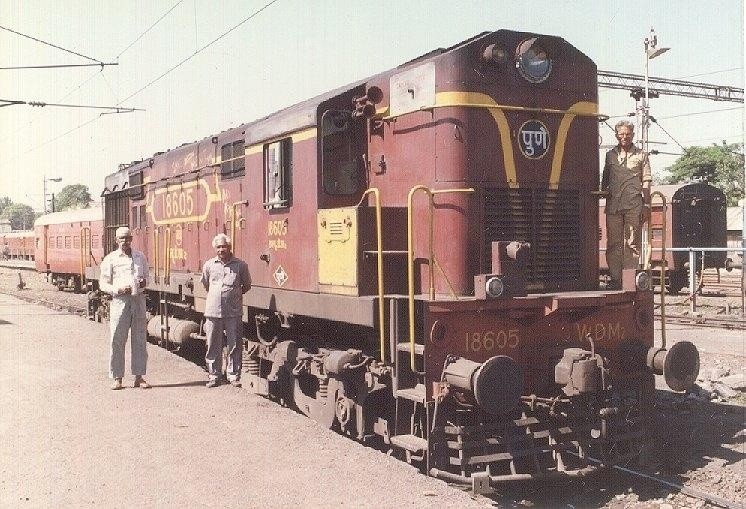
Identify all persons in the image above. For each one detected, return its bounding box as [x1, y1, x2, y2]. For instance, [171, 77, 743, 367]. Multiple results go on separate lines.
[600, 119, 654, 293]
[198, 232, 252, 389]
[98, 226, 152, 389]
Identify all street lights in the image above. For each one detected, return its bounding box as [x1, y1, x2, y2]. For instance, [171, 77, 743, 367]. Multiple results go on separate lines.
[643, 26, 670, 152]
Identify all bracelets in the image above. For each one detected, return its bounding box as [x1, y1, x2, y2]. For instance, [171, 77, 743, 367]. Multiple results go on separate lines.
[644, 203, 652, 209]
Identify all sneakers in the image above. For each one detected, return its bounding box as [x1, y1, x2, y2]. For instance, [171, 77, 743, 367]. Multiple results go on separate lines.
[606, 280, 623, 291]
[132, 380, 152, 389]
[227, 376, 243, 388]
[205, 377, 218, 388]
[110, 378, 122, 390]
